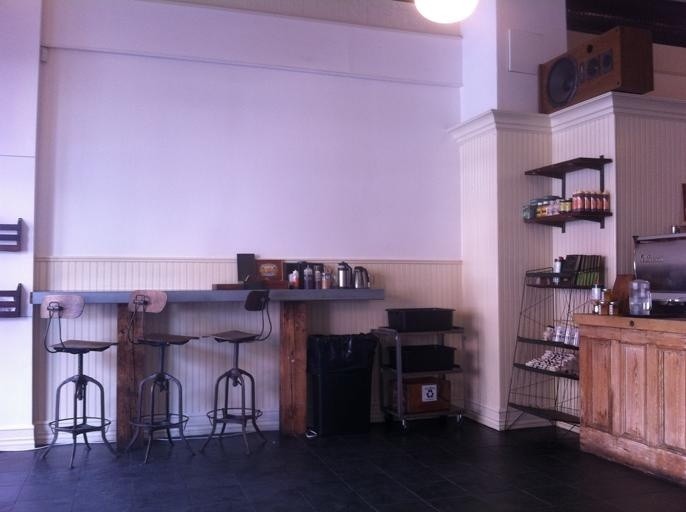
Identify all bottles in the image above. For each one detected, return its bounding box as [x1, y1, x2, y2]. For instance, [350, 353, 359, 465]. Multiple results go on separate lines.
[303, 265, 331, 290]
[591, 283, 618, 316]
[536, 191, 610, 217]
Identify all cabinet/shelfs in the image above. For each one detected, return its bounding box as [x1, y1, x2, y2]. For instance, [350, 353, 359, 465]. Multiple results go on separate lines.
[374, 326, 465, 432]
[523, 154, 613, 234]
[503, 265, 607, 441]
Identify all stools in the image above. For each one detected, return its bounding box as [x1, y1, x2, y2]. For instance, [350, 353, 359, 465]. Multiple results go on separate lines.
[39, 292, 120, 469]
[121, 288, 200, 466]
[199, 290, 271, 457]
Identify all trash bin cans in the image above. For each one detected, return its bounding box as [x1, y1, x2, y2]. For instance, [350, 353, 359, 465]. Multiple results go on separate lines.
[307, 333, 376, 439]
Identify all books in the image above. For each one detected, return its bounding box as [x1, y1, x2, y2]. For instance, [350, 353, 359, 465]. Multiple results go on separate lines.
[558, 254, 601, 286]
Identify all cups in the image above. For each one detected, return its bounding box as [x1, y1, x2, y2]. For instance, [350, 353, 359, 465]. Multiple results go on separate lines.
[628, 280, 652, 316]
[288, 274, 299, 288]
[338, 262, 370, 289]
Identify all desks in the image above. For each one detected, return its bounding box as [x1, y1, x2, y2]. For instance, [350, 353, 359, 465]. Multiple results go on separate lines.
[30, 288, 385, 444]
